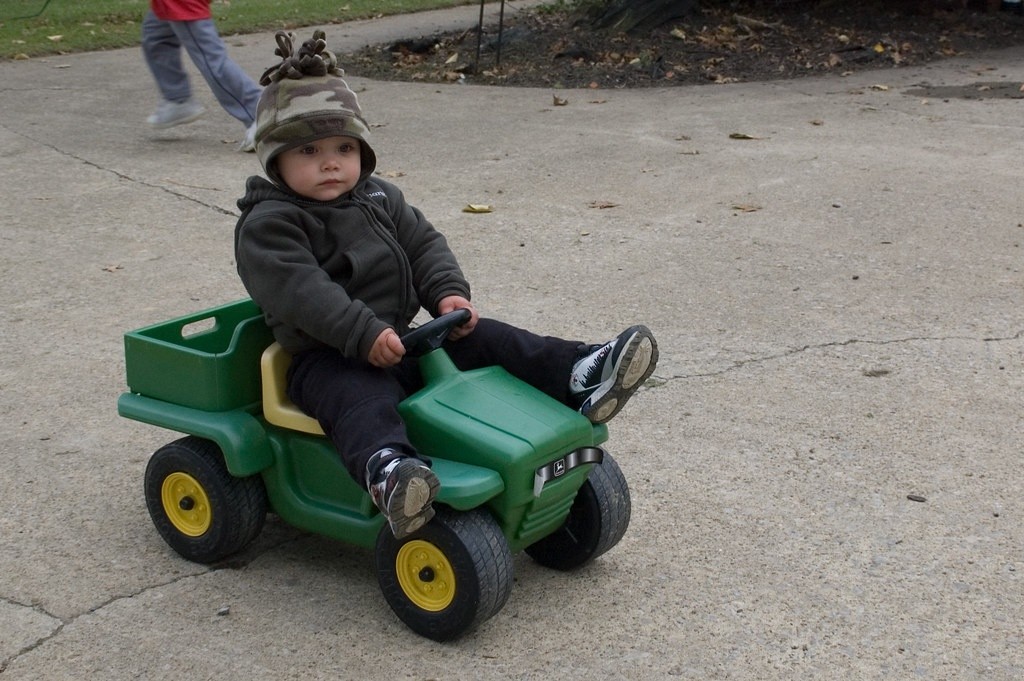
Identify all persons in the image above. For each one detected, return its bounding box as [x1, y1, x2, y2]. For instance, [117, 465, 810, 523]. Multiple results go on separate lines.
[232, 30, 658, 537]
[140, 0, 264, 152]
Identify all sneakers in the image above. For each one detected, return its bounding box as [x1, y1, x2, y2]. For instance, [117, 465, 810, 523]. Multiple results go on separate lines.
[365, 449, 441, 539]
[146, 96, 205, 128]
[243, 122, 257, 153]
[569, 323, 659, 425]
